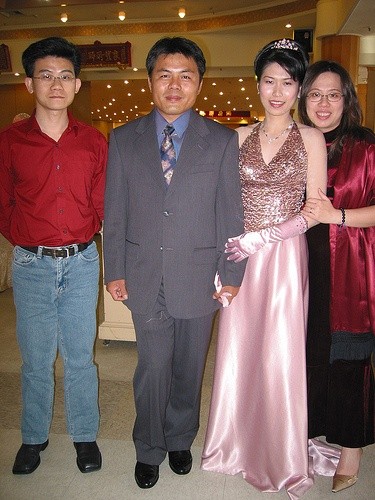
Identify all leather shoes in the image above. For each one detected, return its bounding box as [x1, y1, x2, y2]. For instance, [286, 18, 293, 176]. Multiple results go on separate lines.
[74, 441, 101, 474]
[168, 449, 192, 475]
[12, 439, 48, 475]
[135, 461, 161, 489]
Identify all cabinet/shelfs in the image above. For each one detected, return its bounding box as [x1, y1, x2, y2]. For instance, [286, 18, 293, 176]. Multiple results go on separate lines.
[98, 219, 137, 346]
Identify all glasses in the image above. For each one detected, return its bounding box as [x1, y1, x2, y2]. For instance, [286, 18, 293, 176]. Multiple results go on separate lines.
[305, 92, 345, 102]
[32, 73, 77, 84]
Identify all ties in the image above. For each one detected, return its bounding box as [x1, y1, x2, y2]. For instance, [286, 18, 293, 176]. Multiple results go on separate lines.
[159, 125, 177, 187]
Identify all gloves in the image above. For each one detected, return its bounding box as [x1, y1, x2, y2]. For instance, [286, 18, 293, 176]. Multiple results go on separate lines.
[224, 213, 308, 264]
[214, 271, 232, 308]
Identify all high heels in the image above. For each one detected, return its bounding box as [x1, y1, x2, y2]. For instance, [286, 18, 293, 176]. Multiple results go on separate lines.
[331, 447, 363, 492]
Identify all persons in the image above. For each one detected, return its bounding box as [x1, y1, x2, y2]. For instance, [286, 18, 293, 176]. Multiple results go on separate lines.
[297, 60, 375, 493]
[0, 36, 108, 474]
[104, 37, 248, 488]
[213, 39, 327, 500]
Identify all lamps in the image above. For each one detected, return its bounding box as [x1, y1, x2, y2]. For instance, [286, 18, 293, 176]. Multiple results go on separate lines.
[119, 10, 125, 21]
[178, 8, 186, 19]
[60, 13, 68, 23]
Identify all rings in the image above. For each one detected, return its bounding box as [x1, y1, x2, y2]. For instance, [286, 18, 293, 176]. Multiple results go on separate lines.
[310, 208, 312, 213]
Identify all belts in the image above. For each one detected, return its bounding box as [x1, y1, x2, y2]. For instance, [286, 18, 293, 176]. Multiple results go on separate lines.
[18, 238, 96, 259]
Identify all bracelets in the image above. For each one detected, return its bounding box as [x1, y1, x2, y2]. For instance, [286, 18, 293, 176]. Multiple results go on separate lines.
[337, 207, 345, 227]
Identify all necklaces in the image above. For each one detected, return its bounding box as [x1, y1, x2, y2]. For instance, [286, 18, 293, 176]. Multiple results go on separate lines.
[263, 123, 295, 144]
